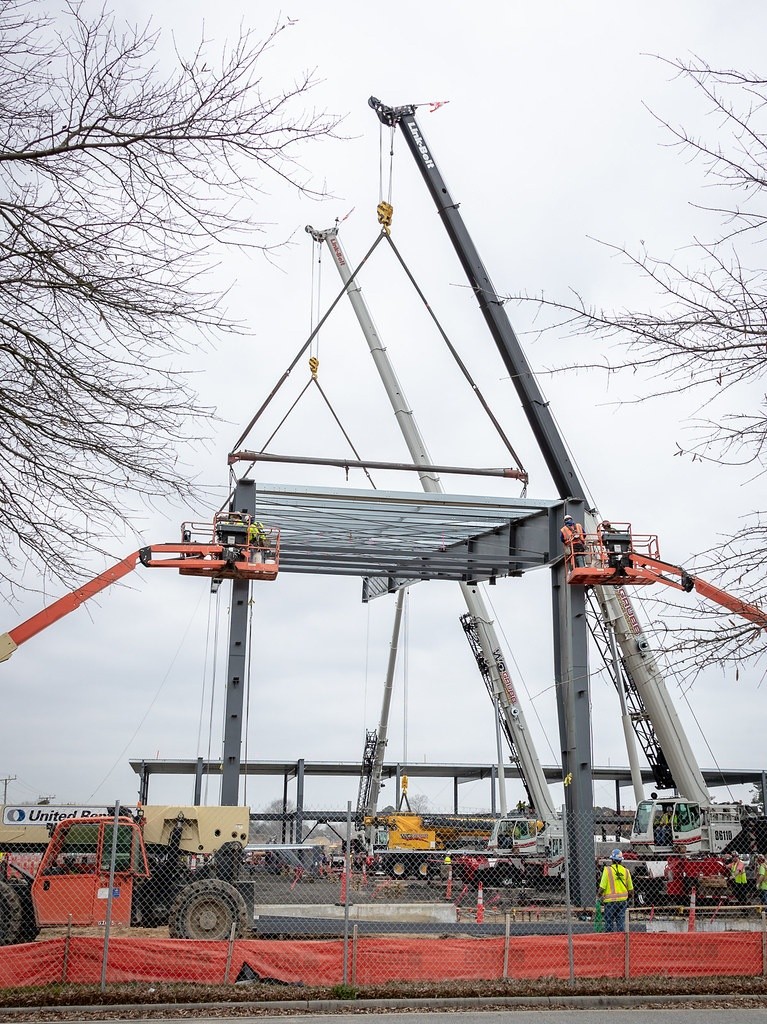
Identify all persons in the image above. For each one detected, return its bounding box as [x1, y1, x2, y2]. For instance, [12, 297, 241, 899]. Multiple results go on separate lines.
[559, 515, 587, 572]
[601, 826, 607, 842]
[595, 848, 634, 933]
[602, 520, 637, 553]
[723, 851, 750, 919]
[655, 807, 677, 846]
[500, 825, 520, 849]
[516, 800, 526, 814]
[755, 853, 767, 916]
[615, 825, 622, 842]
[228, 511, 268, 547]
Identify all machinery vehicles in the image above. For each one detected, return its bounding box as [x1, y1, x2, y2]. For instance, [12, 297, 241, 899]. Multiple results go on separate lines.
[0, 798, 258, 950]
[354, 812, 452, 881]
[300, 95, 767, 921]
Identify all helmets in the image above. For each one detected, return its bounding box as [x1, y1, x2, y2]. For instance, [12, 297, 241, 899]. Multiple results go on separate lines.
[731, 851, 739, 857]
[609, 849, 624, 861]
[232, 511, 242, 519]
[666, 806, 673, 812]
[245, 516, 253, 521]
[758, 854, 765, 861]
